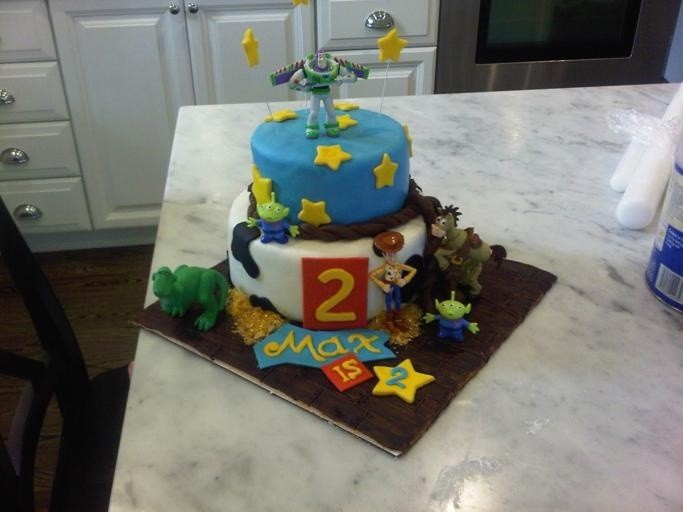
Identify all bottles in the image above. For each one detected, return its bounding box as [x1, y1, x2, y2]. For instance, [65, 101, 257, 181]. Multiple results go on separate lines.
[644, 132, 683, 313]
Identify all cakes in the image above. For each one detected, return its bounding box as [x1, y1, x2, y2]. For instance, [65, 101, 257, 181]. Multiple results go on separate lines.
[226, 50, 442, 323]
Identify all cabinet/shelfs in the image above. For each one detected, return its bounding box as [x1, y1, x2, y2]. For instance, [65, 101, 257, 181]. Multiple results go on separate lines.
[316, 2, 443, 98]
[0, 0, 93, 253]
[43, 1, 315, 245]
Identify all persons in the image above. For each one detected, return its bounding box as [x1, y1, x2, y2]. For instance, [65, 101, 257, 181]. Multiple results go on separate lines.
[369, 230, 417, 333]
[269, 47, 371, 139]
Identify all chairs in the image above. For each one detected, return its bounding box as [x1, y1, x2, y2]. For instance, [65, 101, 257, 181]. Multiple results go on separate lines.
[0, 196, 134, 512]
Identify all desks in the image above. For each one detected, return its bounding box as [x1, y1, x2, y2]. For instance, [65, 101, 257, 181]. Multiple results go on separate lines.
[102, 82, 682, 512]
